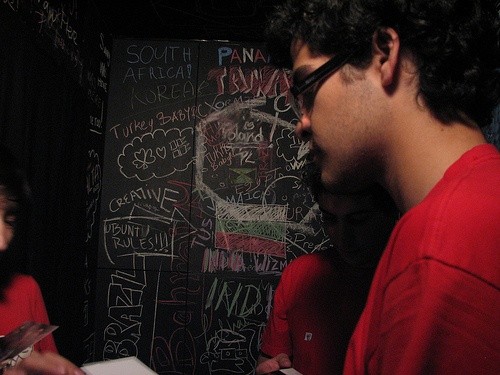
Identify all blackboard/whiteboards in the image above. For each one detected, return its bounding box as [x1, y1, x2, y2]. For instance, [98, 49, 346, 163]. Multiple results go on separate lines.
[94, 38, 333, 375]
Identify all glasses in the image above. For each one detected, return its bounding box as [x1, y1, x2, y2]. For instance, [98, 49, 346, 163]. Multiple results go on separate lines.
[288, 39, 365, 118]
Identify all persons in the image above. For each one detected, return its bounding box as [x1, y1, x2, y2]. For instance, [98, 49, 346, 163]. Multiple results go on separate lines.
[267, 0, 500, 375]
[0, 146, 90, 375]
[255, 164, 402, 375]
